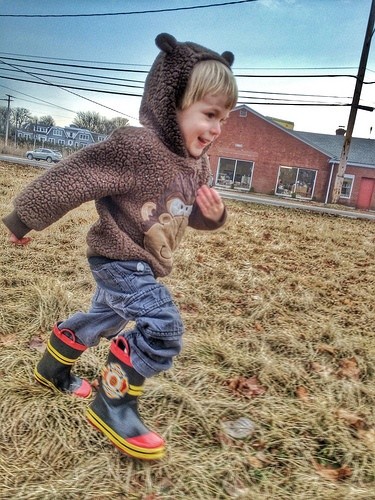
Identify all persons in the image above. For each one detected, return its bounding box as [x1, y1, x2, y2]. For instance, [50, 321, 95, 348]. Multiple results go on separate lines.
[2, 32, 240, 458]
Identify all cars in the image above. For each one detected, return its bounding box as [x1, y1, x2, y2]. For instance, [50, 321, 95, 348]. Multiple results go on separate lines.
[27, 145, 64, 164]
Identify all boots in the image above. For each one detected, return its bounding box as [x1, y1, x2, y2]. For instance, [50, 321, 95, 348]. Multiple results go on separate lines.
[33, 321, 93, 401]
[84, 335, 168, 461]
